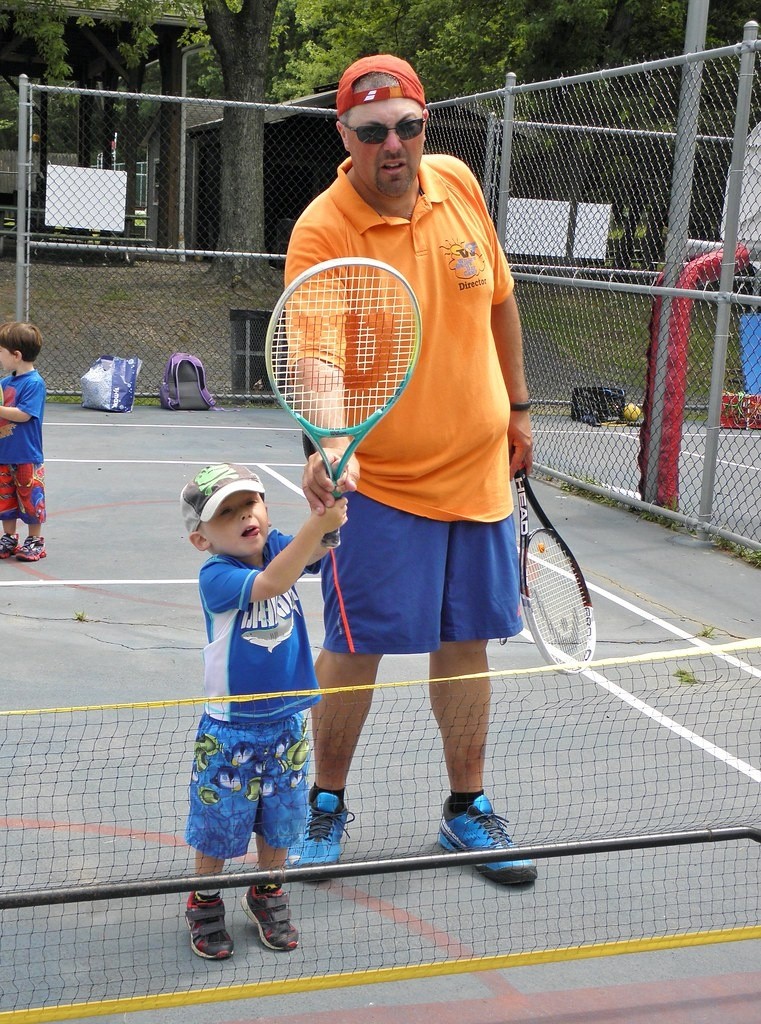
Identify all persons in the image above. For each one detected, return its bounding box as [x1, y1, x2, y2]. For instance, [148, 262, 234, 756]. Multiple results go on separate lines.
[0, 321, 47, 563]
[179, 456, 352, 958]
[281, 54, 539, 886]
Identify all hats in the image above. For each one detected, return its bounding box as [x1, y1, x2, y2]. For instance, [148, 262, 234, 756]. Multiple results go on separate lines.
[336, 53, 427, 110]
[179, 462, 266, 534]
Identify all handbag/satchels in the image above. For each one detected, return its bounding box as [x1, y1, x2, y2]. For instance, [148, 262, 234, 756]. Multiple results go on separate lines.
[81, 356, 143, 413]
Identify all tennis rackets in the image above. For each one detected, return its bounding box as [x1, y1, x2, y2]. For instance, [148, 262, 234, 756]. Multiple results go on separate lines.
[265, 255, 423, 549]
[514, 461, 596, 676]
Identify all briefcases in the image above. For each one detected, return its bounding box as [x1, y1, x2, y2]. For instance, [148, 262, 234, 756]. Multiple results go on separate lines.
[717, 390, 760, 428]
[572, 384, 625, 424]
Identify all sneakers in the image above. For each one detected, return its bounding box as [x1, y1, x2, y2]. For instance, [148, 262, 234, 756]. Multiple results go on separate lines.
[15, 536, 46, 561]
[186, 890, 233, 959]
[239, 881, 300, 952]
[438, 795, 538, 883]
[0, 532, 18, 558]
[288, 793, 349, 866]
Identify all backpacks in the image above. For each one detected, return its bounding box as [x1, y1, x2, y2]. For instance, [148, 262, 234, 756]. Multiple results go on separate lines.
[161, 353, 215, 410]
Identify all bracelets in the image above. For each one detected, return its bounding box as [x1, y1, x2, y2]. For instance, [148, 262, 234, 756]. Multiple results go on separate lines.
[510, 398, 532, 411]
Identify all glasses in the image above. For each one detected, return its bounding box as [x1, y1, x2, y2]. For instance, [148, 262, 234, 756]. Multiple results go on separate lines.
[341, 117, 425, 144]
